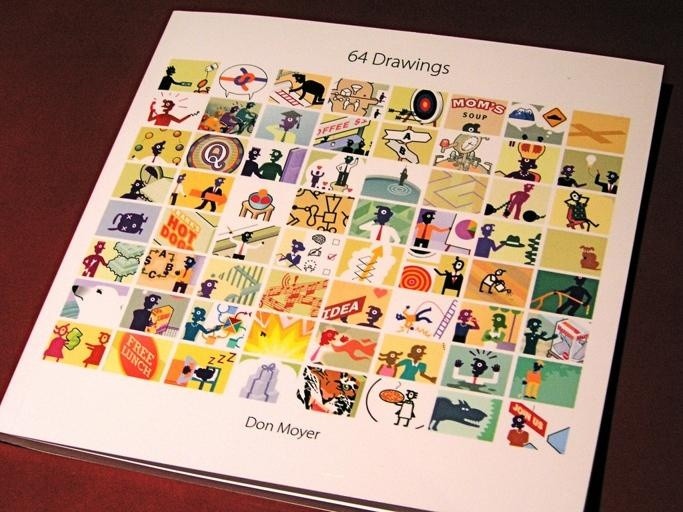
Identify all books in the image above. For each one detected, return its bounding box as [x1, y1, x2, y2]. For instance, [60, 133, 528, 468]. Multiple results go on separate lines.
[0, 12, 664, 512]
